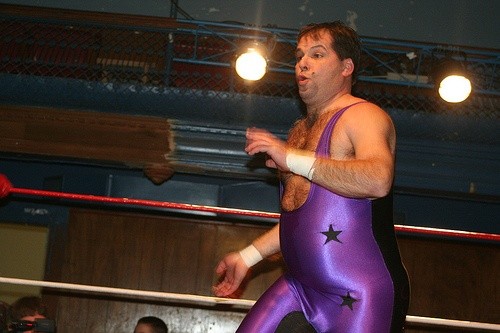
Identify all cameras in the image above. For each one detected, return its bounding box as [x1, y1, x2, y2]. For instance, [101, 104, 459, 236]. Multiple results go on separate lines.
[14, 319, 55, 333]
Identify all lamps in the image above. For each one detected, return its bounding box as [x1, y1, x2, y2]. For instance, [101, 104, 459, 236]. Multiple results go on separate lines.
[230, 27, 474, 105]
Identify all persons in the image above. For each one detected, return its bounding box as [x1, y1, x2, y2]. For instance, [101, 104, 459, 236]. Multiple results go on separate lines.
[7, 296, 50, 333]
[132, 315, 168, 333]
[211, 20, 410, 333]
